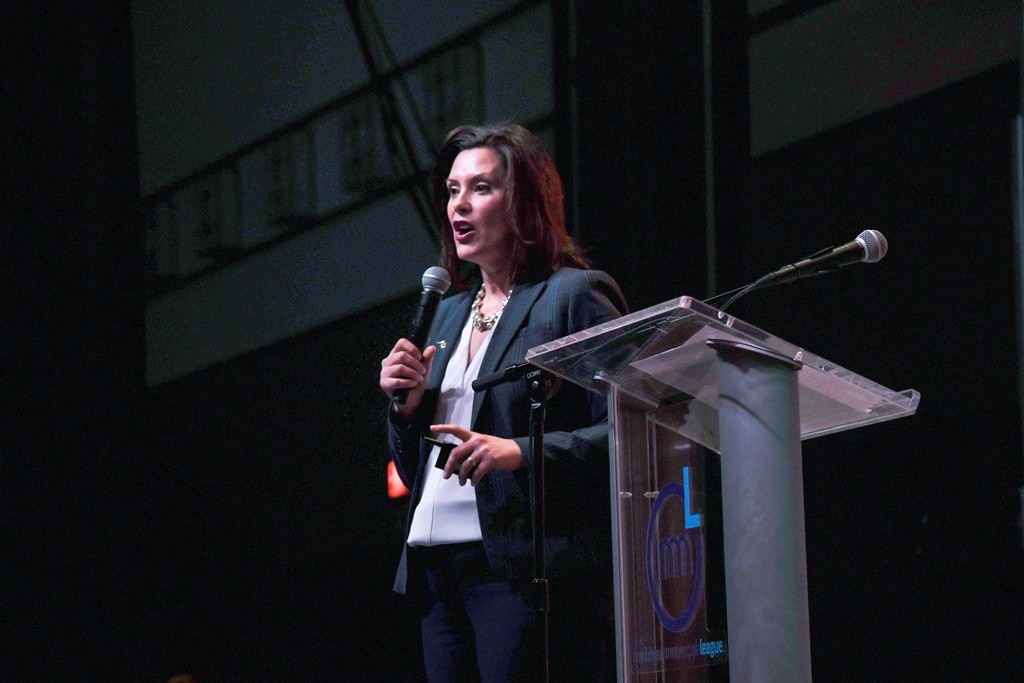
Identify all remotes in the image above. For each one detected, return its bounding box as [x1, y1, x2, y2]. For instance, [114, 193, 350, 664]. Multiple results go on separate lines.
[424, 437, 478, 478]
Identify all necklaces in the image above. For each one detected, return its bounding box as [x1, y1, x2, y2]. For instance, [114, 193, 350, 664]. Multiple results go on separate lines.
[471, 283, 513, 332]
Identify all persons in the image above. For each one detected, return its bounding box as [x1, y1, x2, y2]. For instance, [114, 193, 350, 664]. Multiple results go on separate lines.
[380, 124, 644, 683]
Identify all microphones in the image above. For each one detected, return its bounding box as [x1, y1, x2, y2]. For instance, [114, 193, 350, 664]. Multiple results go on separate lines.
[394, 266, 451, 406]
[750, 229, 888, 290]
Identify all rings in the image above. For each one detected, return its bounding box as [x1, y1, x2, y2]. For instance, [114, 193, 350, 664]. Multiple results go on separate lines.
[468, 457, 476, 467]
